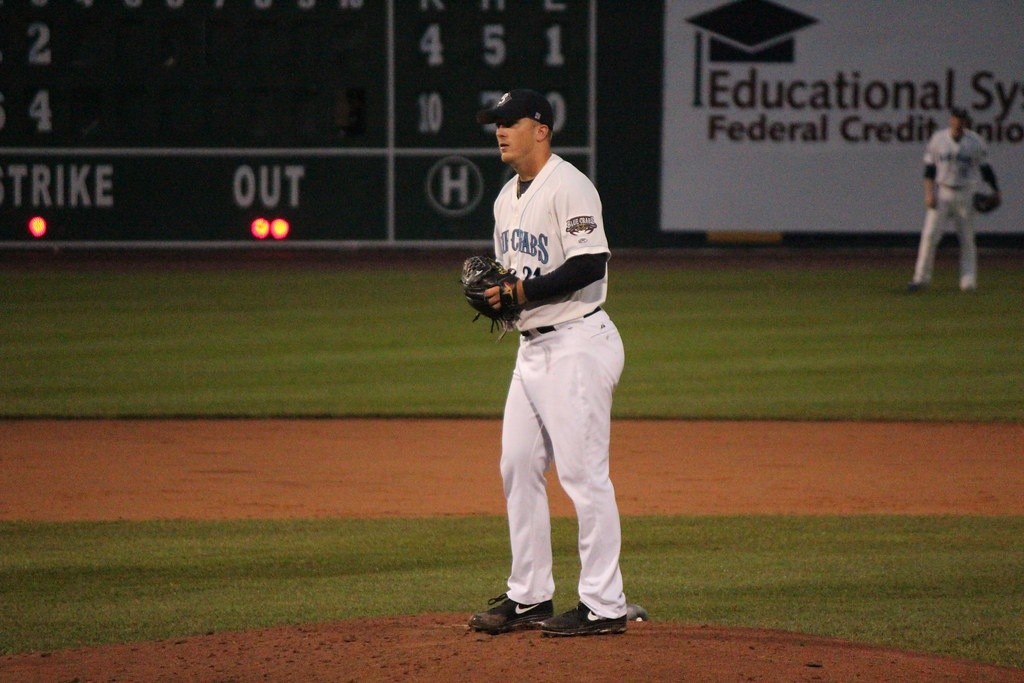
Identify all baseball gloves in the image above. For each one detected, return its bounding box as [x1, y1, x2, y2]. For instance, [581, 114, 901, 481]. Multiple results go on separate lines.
[972, 191, 1001, 215]
[460, 254, 509, 318]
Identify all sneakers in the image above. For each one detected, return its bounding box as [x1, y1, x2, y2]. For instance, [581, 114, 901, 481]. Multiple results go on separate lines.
[539, 602, 628, 637]
[468, 599, 554, 634]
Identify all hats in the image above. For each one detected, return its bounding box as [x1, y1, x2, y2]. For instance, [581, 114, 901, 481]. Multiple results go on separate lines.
[477, 88, 554, 131]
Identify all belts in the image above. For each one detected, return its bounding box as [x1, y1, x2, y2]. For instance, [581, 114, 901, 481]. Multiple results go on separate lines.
[521, 306, 602, 339]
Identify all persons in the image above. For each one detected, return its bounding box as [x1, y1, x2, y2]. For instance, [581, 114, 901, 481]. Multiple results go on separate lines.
[906, 106, 1002, 296]
[459, 89, 627, 637]
